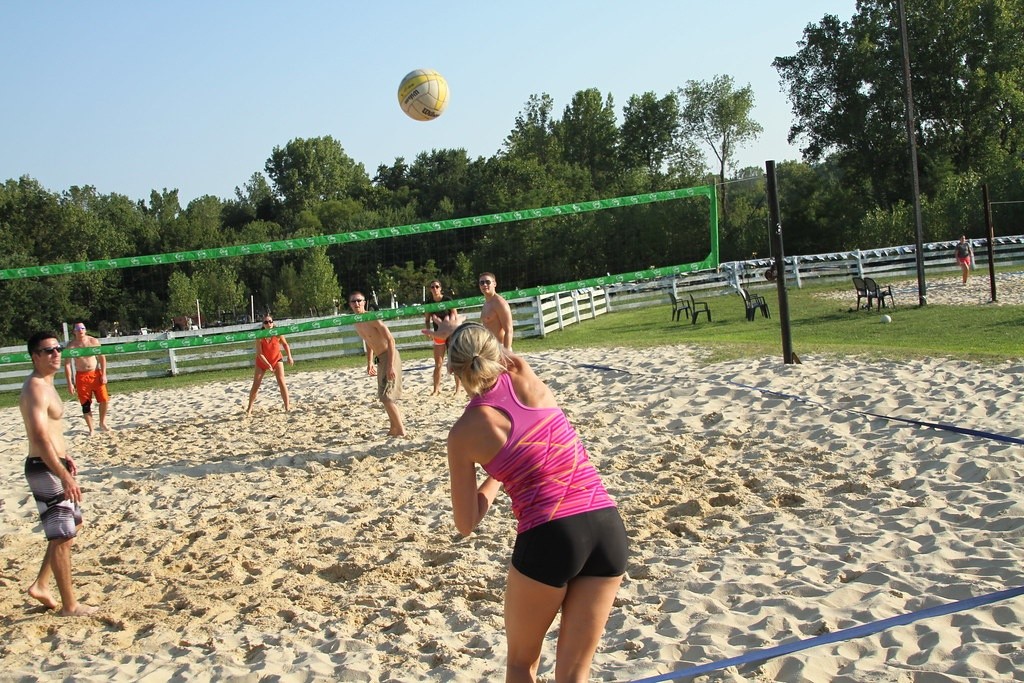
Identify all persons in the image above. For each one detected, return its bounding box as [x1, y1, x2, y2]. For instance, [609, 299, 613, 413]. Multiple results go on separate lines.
[19, 331, 100, 617]
[246, 313, 293, 413]
[416, 310, 628, 683]
[423, 280, 460, 395]
[64, 323, 108, 432]
[477, 272, 513, 354]
[955, 235, 973, 285]
[349, 291, 404, 439]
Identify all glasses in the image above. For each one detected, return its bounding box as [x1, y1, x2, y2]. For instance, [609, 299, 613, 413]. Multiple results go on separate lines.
[35, 345, 62, 354]
[431, 285, 440, 288]
[264, 320, 273, 324]
[480, 280, 493, 284]
[73, 326, 86, 331]
[351, 299, 363, 303]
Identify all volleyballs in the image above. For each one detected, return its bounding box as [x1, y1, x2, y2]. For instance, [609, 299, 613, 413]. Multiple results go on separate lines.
[397, 68, 450, 122]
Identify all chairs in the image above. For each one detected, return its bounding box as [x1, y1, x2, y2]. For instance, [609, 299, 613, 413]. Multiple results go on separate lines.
[668, 292, 692, 322]
[689, 293, 712, 324]
[852, 277, 895, 311]
[739, 289, 772, 321]
